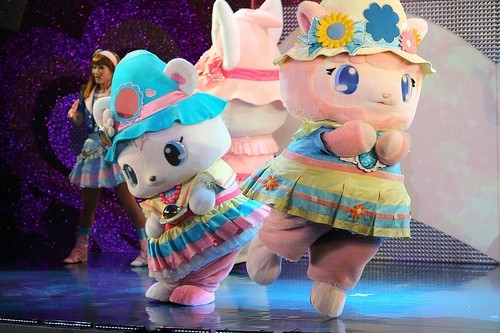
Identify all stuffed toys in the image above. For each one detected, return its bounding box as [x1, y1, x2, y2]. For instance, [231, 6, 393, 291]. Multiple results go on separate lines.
[237, 1, 437, 317]
[194, 0, 287, 266]
[93, 50, 273, 306]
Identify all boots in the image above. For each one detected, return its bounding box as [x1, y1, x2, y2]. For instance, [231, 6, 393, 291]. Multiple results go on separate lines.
[64, 226, 92, 263]
[130, 228, 148, 266]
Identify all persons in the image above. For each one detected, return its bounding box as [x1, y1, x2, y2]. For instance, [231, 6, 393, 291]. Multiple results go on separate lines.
[64, 49, 146, 266]
